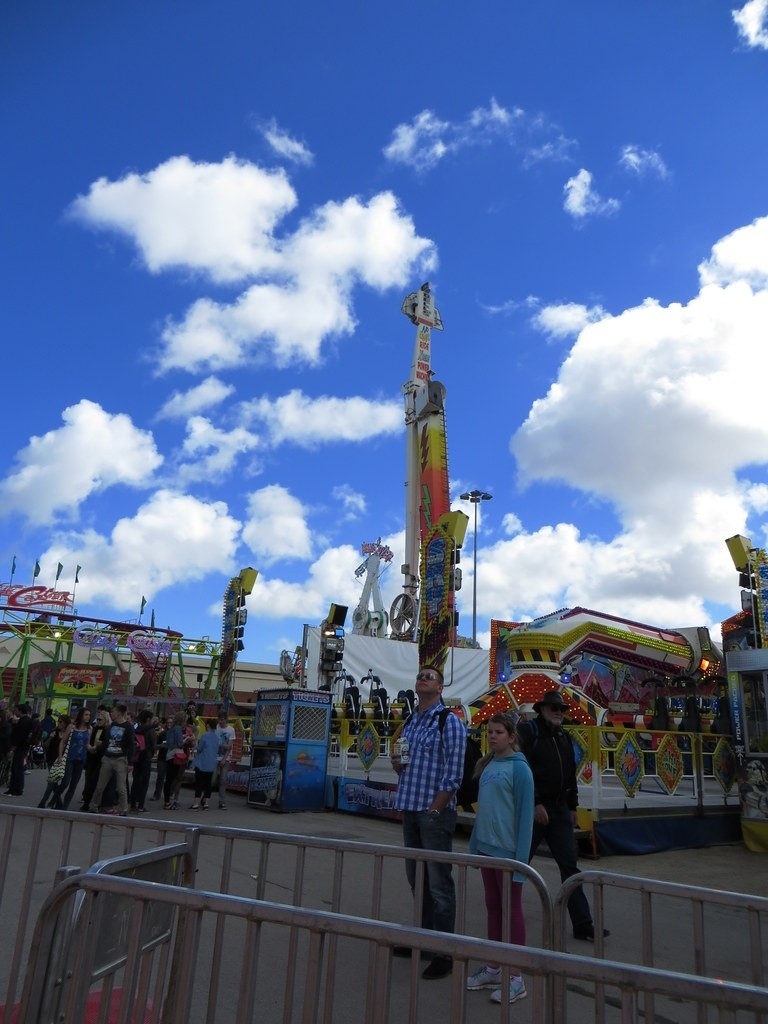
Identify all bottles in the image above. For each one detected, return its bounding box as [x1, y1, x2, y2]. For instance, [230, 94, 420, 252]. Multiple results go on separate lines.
[107, 747, 115, 753]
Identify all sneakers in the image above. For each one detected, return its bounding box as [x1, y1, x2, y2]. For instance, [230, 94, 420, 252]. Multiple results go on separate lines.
[130, 808, 151, 815]
[80, 804, 90, 810]
[219, 802, 227, 810]
[490, 976, 527, 1003]
[422, 954, 454, 980]
[119, 809, 127, 816]
[202, 805, 208, 813]
[2, 790, 24, 798]
[38, 803, 46, 808]
[148, 797, 161, 804]
[164, 803, 178, 810]
[88, 806, 98, 813]
[393, 939, 432, 960]
[467, 965, 502, 989]
[187, 804, 199, 813]
[24, 769, 31, 774]
[573, 921, 611, 939]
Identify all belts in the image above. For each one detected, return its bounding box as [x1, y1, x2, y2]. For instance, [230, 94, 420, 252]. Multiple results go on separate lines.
[105, 750, 124, 758]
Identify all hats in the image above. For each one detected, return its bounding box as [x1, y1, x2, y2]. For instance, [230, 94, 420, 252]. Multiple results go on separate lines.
[532, 692, 571, 712]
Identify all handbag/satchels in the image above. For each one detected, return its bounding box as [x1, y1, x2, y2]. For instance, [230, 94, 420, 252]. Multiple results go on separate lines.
[33, 744, 43, 754]
[174, 753, 187, 765]
[47, 727, 73, 783]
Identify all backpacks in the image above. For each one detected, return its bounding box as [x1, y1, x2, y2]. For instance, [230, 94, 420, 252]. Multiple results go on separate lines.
[132, 733, 145, 763]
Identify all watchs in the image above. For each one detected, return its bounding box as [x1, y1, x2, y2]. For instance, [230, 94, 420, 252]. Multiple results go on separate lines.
[429, 809, 440, 819]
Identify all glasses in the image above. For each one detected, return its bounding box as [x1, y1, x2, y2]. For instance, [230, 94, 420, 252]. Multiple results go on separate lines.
[416, 673, 441, 680]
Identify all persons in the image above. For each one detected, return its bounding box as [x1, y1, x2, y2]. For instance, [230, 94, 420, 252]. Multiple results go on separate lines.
[467, 711, 534, 1003]
[0, 700, 236, 816]
[711, 698, 732, 743]
[516, 691, 610, 941]
[678, 697, 701, 752]
[648, 697, 670, 731]
[390, 665, 468, 979]
